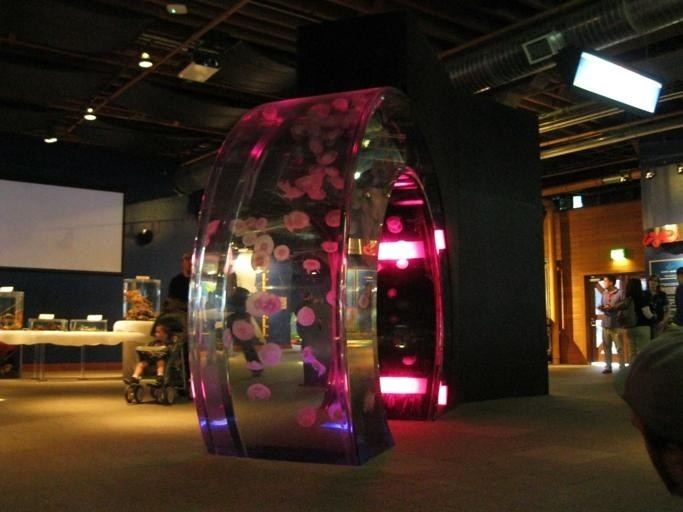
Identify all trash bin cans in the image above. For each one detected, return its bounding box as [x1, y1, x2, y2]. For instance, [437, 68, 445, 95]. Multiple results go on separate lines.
[546, 317, 552, 364]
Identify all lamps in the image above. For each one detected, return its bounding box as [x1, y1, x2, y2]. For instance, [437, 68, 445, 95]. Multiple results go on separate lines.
[558, 47, 665, 120]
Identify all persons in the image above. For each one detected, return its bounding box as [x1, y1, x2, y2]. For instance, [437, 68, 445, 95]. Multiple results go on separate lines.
[596, 267, 683, 374]
[610, 329, 683, 499]
[164, 250, 200, 312]
[222, 272, 264, 379]
[122, 321, 176, 389]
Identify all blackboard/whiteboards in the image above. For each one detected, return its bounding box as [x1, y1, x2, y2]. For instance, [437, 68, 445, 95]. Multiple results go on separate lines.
[0, 178, 125, 275]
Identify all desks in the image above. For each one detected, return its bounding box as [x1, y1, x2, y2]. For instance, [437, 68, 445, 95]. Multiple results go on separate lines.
[0, 328, 146, 382]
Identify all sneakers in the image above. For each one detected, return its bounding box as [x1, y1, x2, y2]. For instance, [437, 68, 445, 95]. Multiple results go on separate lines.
[156, 376, 164, 384]
[123, 377, 139, 384]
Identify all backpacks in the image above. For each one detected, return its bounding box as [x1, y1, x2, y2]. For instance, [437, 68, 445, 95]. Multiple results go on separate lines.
[617, 297, 637, 327]
